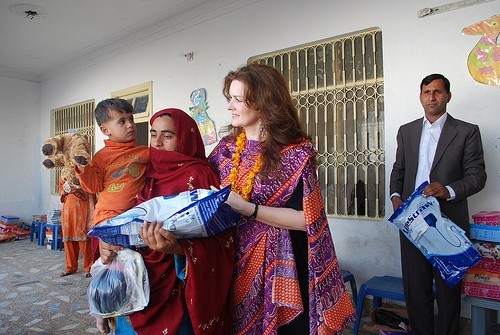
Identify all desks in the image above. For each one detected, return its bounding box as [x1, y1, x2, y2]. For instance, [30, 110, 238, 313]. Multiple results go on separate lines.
[462, 296, 500, 335]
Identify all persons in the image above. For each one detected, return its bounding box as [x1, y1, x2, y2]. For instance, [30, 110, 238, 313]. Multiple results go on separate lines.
[209, 64, 359, 335]
[99, 108, 236, 335]
[389, 73, 487, 335]
[72, 97, 149, 335]
[59, 168, 99, 278]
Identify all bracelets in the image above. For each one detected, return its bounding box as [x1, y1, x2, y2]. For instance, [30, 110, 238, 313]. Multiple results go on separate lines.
[250, 203, 258, 219]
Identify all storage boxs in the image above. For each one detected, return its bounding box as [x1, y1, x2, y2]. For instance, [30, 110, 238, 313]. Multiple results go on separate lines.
[45, 231, 53, 240]
[0, 221, 17, 229]
[463, 242, 500, 300]
[47, 242, 64, 250]
[471, 208, 500, 226]
[469, 224, 500, 243]
[1, 215, 20, 223]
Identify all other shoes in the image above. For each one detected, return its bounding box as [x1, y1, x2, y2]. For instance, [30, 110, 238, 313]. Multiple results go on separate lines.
[60, 271, 72, 276]
[85, 273, 91, 278]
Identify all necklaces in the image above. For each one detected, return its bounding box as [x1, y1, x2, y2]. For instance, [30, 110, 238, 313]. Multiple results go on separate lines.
[230, 130, 263, 200]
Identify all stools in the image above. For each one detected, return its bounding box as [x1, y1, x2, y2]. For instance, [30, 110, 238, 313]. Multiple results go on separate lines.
[341, 267, 358, 306]
[40, 222, 64, 251]
[29, 220, 41, 244]
[352, 275, 410, 335]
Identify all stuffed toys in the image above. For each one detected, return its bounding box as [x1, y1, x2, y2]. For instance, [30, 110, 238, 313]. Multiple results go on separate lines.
[42, 133, 90, 167]
